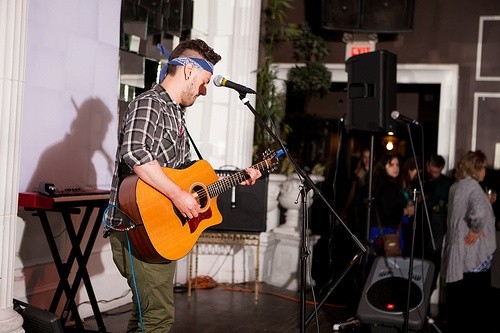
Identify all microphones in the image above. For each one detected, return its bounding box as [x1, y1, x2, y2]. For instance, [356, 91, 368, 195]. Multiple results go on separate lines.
[391, 111, 418, 126]
[213, 75, 257, 94]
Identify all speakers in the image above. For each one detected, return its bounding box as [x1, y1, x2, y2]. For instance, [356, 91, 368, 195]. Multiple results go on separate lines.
[205, 170, 269, 232]
[344, 49, 398, 133]
[146, 0, 194, 37]
[357, 256, 435, 332]
[321, 0, 361, 32]
[361, 0, 415, 33]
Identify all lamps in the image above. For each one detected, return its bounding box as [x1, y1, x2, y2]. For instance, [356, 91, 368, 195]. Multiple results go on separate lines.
[342, 32, 378, 60]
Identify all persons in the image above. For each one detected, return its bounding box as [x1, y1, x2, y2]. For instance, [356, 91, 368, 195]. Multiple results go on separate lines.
[409, 155, 452, 267]
[339, 149, 375, 237]
[103, 39, 262, 333]
[442, 150, 497, 333]
[369, 154, 414, 257]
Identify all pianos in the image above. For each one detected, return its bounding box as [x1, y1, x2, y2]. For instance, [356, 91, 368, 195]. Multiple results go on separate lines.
[18, 185, 111, 333]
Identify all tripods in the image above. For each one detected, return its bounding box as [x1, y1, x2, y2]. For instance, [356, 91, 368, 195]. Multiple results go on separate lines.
[298, 135, 375, 327]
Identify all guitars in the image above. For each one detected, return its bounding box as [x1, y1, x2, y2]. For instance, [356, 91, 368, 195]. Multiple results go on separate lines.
[118, 148, 281, 263]
